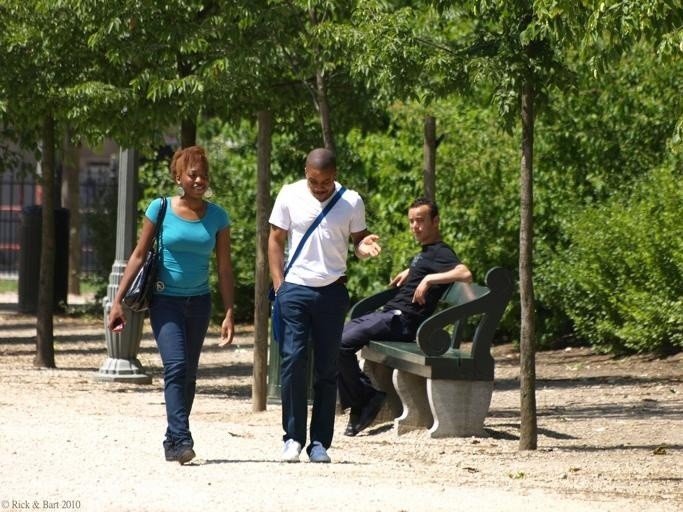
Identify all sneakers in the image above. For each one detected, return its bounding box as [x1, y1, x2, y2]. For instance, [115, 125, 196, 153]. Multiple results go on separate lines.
[345, 388, 386, 436]
[309, 441, 331, 463]
[164, 447, 195, 465]
[282, 438, 301, 461]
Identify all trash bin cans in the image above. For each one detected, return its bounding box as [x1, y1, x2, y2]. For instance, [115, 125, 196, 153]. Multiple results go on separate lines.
[18, 205, 71, 314]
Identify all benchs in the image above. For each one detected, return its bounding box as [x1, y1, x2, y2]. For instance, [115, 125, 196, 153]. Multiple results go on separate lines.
[358, 266, 512, 440]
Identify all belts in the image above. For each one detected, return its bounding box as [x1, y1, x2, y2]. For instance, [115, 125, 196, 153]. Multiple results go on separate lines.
[388, 308, 405, 314]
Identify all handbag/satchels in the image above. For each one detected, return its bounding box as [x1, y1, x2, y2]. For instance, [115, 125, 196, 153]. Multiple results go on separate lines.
[270, 296, 284, 343]
[122, 195, 166, 312]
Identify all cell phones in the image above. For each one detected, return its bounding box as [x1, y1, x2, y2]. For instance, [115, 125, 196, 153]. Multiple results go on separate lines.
[112, 319, 123, 332]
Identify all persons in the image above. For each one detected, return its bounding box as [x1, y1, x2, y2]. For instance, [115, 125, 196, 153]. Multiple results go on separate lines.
[266, 147, 381, 465]
[103, 147, 235, 466]
[336, 197, 474, 437]
